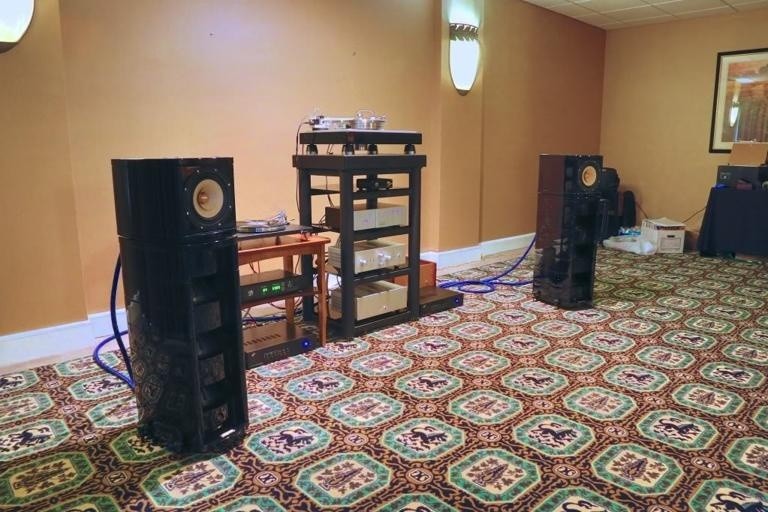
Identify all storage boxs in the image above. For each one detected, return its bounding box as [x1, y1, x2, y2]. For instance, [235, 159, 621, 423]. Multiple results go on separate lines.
[640, 216, 686, 254]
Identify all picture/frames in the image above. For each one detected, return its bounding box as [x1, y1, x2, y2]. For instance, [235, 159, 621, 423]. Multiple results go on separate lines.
[709, 48, 768, 153]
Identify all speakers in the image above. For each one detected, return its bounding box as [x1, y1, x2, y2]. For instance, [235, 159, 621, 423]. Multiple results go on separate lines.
[533, 154, 603, 309]
[110, 156, 249, 454]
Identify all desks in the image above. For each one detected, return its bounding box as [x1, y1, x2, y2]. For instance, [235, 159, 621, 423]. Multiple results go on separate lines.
[236, 232, 332, 347]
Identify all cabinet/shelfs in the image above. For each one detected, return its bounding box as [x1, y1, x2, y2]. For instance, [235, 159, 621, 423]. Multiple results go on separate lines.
[292, 152, 427, 339]
